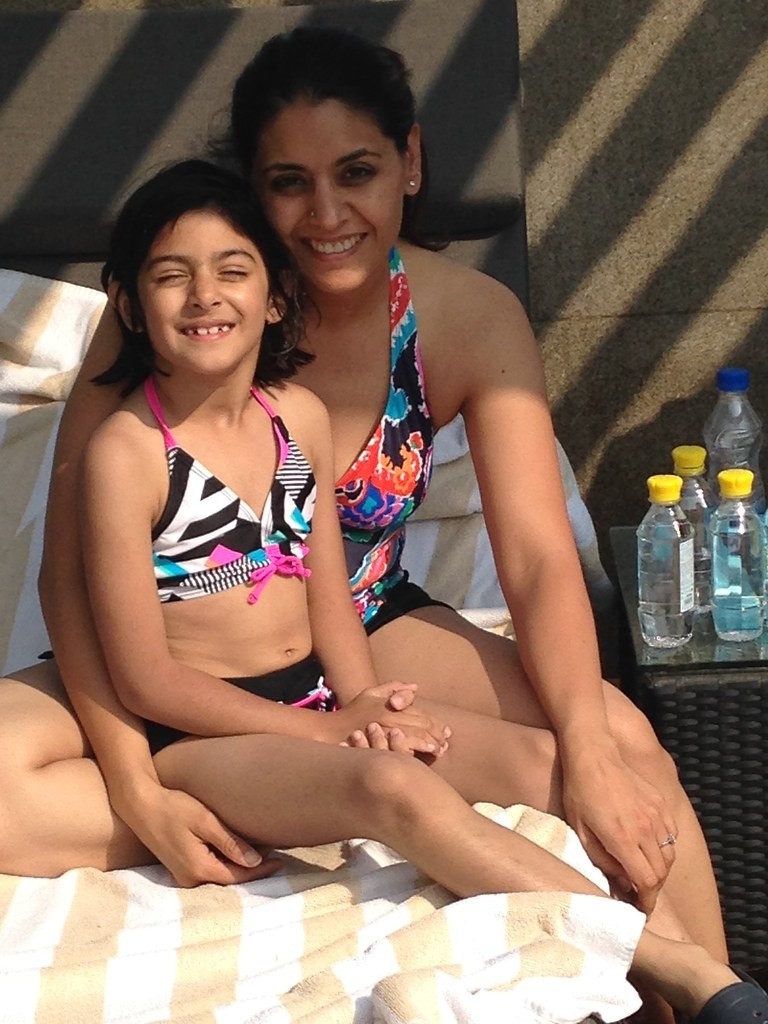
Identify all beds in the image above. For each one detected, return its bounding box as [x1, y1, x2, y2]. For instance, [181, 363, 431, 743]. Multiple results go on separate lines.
[0, 267, 650, 1024]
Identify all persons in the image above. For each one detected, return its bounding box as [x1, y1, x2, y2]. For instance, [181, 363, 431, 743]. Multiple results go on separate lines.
[74, 157, 767, 1024]
[0, 29, 730, 1024]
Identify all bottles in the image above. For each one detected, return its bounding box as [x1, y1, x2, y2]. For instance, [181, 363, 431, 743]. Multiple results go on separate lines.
[635, 473, 695, 648]
[670, 445, 714, 615]
[702, 363, 760, 471]
[708, 470, 768, 641]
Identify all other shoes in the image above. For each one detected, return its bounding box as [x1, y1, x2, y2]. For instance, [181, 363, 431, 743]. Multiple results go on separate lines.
[673, 964, 768, 1024]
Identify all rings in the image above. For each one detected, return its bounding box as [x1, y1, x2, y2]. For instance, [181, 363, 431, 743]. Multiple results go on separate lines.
[659, 834, 677, 848]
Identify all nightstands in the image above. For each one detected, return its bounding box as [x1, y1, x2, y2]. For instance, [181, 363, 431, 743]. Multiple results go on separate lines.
[608, 524, 768, 994]
